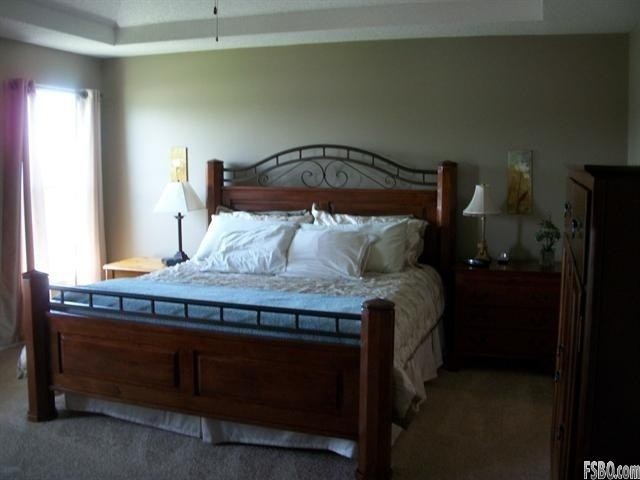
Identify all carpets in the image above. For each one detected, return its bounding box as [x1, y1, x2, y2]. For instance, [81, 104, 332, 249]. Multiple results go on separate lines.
[1, 385, 358, 479]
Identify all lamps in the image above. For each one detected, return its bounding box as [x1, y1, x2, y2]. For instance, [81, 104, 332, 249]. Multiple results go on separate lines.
[156, 182, 206, 267]
[462, 183, 503, 265]
[169, 145, 188, 183]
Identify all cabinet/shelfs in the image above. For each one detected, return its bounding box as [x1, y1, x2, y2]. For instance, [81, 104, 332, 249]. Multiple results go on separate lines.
[550, 163, 640, 480]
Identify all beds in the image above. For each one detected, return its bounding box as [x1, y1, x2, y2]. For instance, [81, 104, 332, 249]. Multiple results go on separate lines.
[17, 144, 458, 480]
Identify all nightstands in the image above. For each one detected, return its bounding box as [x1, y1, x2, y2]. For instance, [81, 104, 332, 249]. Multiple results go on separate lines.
[102, 257, 165, 280]
[442, 255, 562, 372]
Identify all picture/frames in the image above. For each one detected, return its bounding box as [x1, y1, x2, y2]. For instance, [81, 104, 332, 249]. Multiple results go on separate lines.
[506, 148, 533, 215]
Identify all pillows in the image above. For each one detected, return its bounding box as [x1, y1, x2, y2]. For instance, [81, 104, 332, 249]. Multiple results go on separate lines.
[232, 212, 313, 225]
[286, 230, 379, 281]
[199, 214, 299, 274]
[311, 202, 429, 269]
[298, 217, 409, 273]
[195, 215, 288, 261]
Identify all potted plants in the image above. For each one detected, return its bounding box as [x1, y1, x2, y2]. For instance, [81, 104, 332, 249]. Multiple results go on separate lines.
[535, 220, 561, 267]
